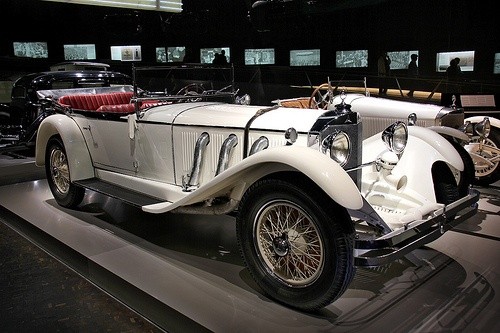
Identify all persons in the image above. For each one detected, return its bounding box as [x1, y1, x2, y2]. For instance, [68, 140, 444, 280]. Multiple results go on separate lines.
[213, 50, 227, 65]
[441, 57, 462, 108]
[378, 53, 391, 96]
[407, 54, 418, 96]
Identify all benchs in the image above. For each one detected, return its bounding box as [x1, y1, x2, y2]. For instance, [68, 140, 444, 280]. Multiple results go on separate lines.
[60, 92, 133, 111]
[95, 100, 171, 113]
[281, 97, 317, 109]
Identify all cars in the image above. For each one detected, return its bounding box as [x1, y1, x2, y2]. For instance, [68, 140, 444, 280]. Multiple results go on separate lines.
[35, 60, 500, 315]
[0, 69, 133, 159]
